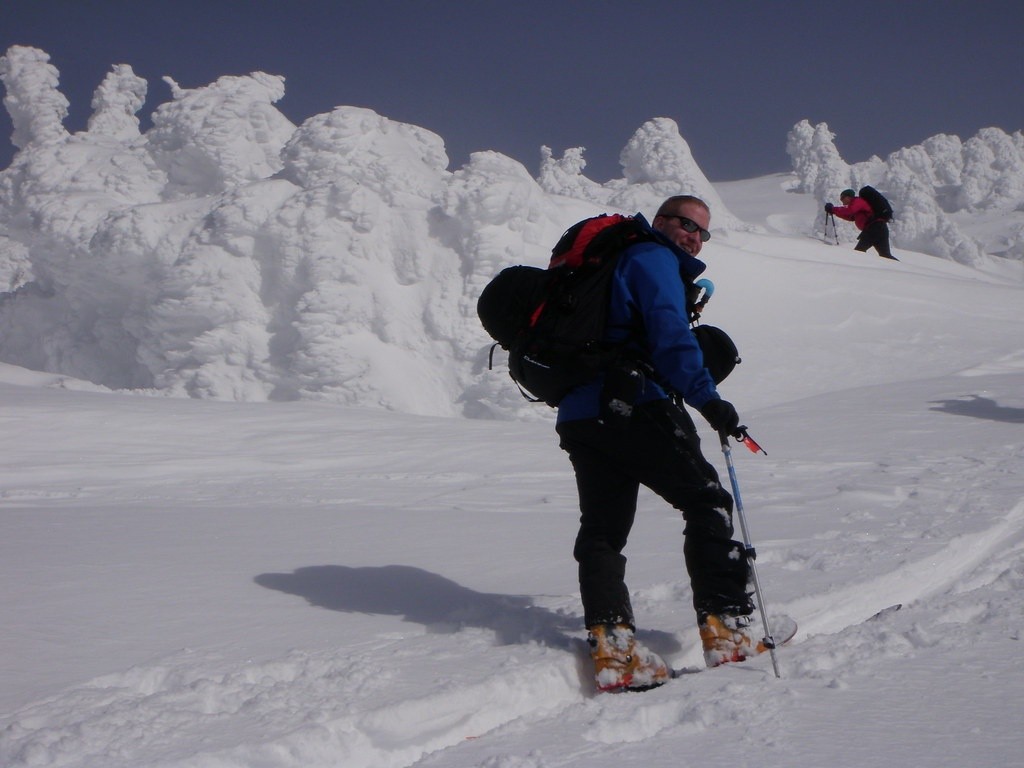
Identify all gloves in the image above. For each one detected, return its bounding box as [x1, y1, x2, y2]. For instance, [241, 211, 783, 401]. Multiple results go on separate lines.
[825, 203, 833, 213]
[701, 398, 739, 436]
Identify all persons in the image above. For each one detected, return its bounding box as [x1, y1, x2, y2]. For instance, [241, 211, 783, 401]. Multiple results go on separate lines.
[554, 194, 799, 692]
[824, 189, 899, 261]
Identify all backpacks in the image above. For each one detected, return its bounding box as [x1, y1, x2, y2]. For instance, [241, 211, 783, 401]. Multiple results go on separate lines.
[859, 186, 892, 223]
[478, 212, 679, 411]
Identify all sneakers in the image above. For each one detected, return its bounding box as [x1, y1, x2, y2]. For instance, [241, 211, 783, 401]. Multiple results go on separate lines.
[698, 611, 797, 668]
[588, 624, 675, 694]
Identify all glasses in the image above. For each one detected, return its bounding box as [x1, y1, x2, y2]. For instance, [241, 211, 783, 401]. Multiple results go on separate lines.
[656, 214, 711, 242]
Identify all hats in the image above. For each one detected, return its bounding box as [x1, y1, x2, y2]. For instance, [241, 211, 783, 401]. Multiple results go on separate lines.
[841, 189, 855, 197]
